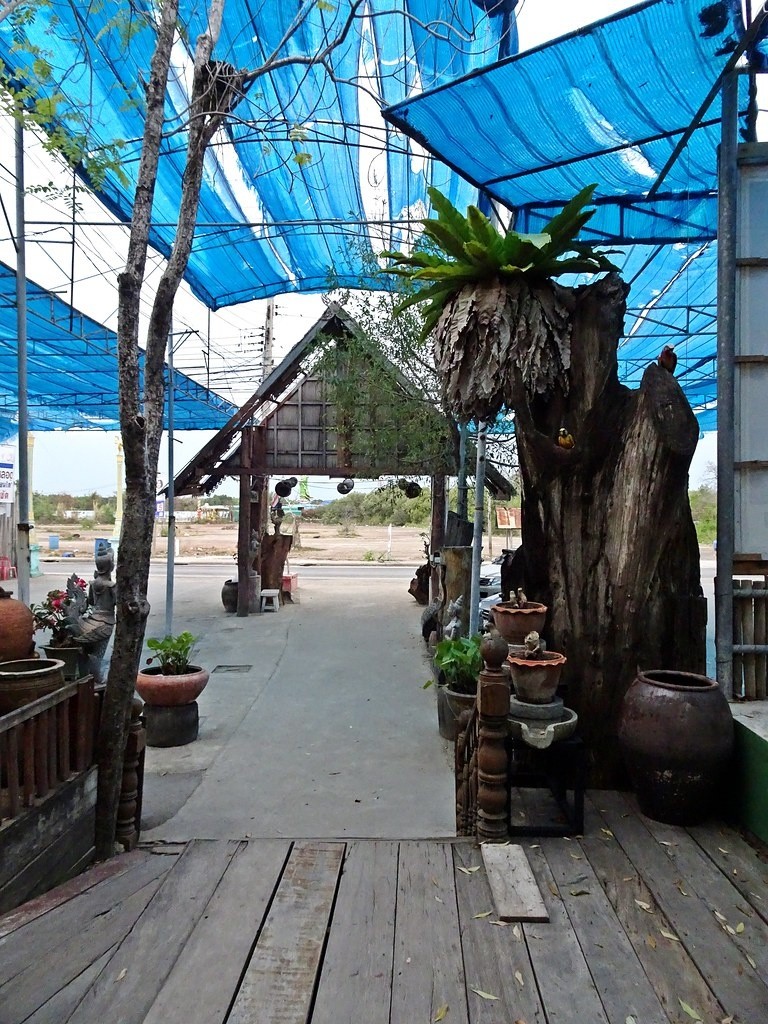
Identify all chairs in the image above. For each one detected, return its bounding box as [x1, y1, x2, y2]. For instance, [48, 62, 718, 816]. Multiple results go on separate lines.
[0, 556, 17, 581]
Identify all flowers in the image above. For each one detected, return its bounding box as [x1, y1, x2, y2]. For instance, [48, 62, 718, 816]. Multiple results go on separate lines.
[29, 573, 88, 648]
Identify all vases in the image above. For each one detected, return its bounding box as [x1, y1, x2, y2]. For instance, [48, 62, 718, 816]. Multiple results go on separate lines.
[38, 644, 79, 681]
[0, 658, 66, 716]
[489, 601, 548, 646]
[506, 651, 566, 704]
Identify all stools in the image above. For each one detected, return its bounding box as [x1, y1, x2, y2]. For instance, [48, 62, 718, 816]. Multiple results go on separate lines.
[260, 589, 281, 613]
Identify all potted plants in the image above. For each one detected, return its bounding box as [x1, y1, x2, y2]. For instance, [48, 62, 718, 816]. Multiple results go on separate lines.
[423, 632, 485, 741]
[135, 632, 209, 708]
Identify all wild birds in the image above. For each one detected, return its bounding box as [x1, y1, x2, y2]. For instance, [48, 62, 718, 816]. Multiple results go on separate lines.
[559, 428, 574, 449]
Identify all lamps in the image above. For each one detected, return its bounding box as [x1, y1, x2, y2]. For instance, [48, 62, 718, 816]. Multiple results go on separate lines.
[399, 478, 422, 499]
[337, 479, 354, 495]
[275, 477, 297, 497]
